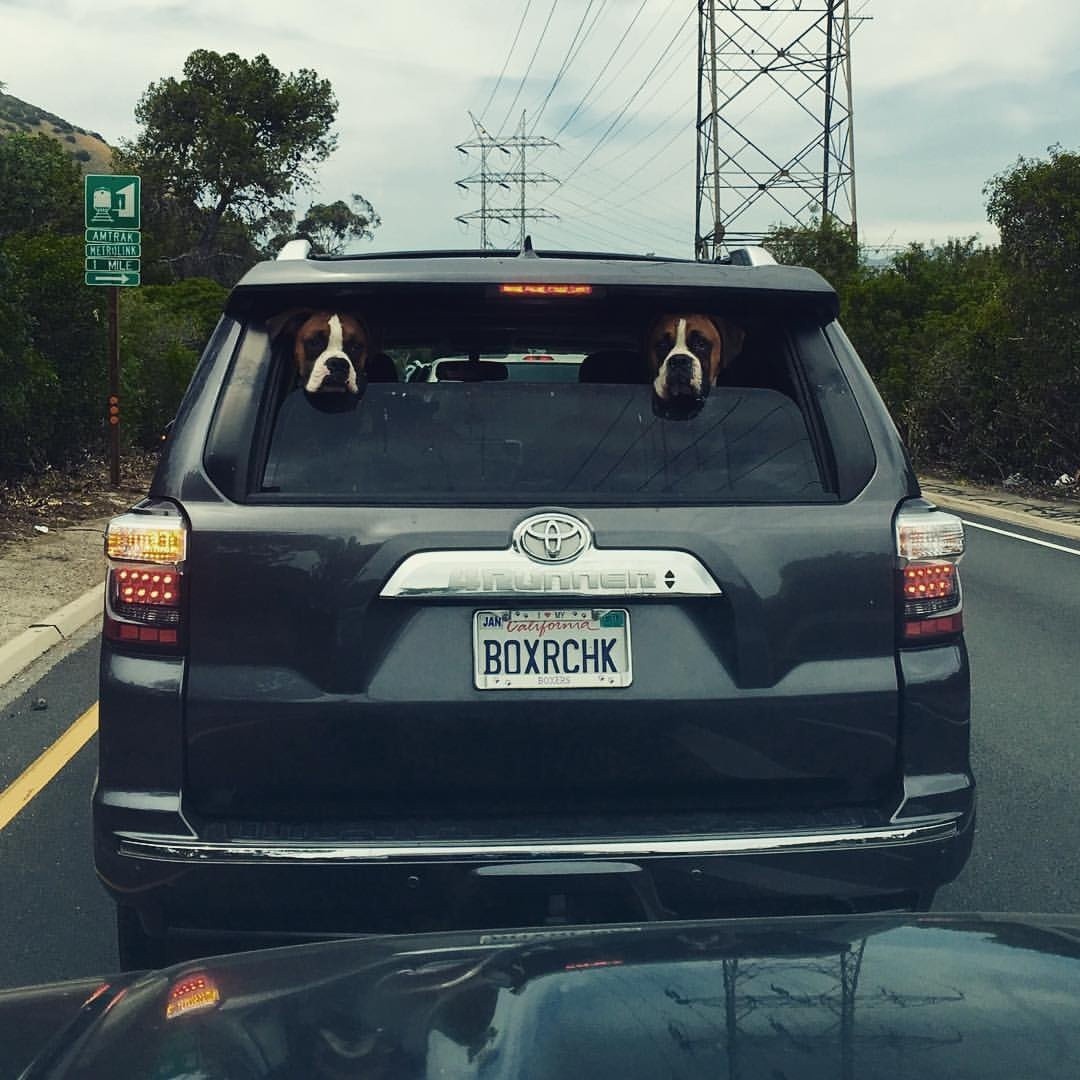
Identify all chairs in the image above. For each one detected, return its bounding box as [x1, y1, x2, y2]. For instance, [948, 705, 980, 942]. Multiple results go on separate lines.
[580, 350, 649, 384]
[367, 353, 399, 382]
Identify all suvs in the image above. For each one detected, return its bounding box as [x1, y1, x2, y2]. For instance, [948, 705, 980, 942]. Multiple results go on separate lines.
[90, 239, 977, 969]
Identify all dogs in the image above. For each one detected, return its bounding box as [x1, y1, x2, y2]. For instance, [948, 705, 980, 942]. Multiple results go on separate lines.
[295, 309, 375, 395]
[645, 313, 742, 407]
